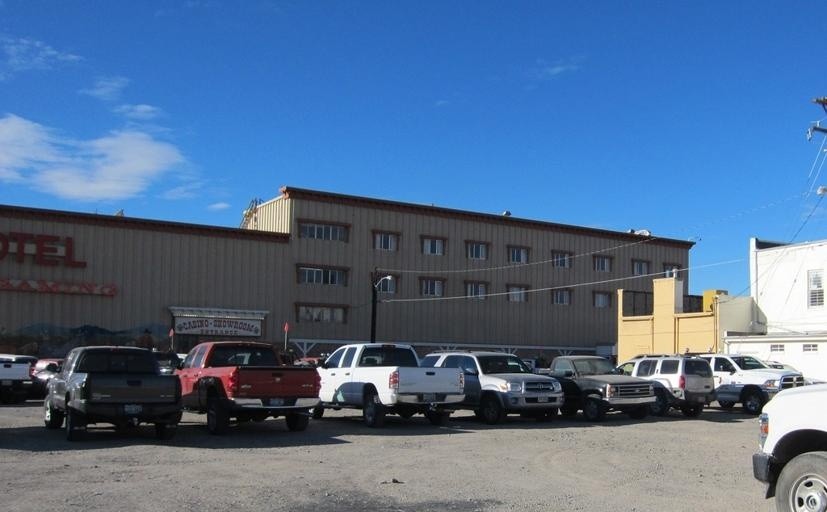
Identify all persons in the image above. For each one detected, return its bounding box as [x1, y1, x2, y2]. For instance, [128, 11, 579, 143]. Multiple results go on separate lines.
[136, 328, 152, 348]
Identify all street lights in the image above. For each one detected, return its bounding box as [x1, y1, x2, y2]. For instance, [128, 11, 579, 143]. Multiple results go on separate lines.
[370, 275, 392, 342]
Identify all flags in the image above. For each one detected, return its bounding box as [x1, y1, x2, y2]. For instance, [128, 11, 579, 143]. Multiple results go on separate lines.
[283, 322, 289, 332]
[168, 329, 174, 337]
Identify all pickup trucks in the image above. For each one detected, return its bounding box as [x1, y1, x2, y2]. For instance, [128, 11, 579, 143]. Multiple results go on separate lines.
[174, 339, 323, 435]
[0, 360, 32, 405]
[43, 345, 183, 442]
[313, 343, 467, 427]
[534, 355, 657, 423]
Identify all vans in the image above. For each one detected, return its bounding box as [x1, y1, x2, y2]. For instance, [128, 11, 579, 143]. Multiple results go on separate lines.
[31, 358, 65, 388]
[0, 353, 38, 374]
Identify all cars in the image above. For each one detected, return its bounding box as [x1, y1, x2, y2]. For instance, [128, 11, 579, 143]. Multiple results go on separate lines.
[718, 360, 826, 410]
[294, 354, 327, 367]
[152, 350, 181, 376]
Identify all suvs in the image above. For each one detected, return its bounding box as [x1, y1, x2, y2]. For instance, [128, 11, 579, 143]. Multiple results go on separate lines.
[611, 355, 717, 419]
[686, 352, 805, 415]
[752, 383, 827, 512]
[420, 348, 567, 425]
